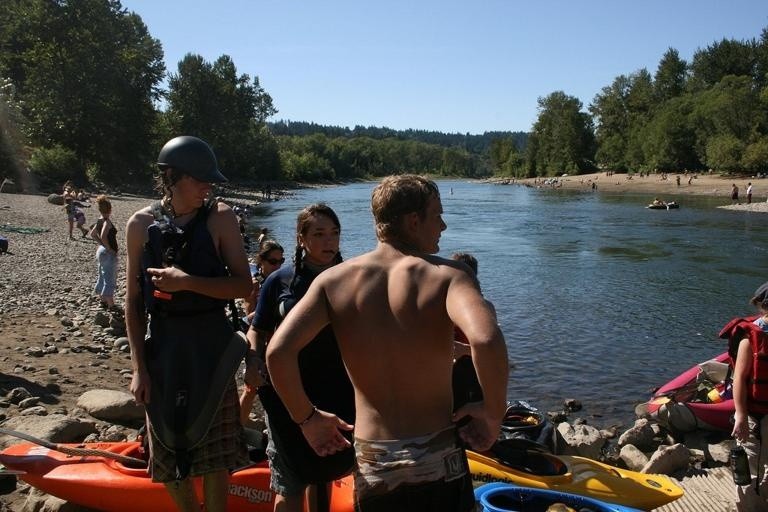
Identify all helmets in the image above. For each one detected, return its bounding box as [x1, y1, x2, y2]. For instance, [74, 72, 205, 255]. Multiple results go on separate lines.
[155, 134, 229, 185]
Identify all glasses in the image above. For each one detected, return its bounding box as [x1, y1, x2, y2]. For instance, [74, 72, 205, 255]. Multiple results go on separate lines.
[258, 253, 285, 265]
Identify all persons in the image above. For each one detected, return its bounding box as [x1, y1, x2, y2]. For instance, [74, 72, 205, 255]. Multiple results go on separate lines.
[719, 282, 768, 512]
[91, 194, 118, 307]
[64, 189, 90, 237]
[535, 168, 766, 206]
[125, 138, 253, 512]
[245, 175, 509, 512]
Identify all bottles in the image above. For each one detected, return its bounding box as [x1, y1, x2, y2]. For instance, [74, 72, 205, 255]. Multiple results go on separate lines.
[729, 441, 752, 486]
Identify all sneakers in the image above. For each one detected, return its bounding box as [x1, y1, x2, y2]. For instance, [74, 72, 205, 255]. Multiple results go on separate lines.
[99, 300, 122, 313]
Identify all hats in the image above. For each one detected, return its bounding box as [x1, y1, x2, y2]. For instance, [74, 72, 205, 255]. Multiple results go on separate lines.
[751, 280, 767, 302]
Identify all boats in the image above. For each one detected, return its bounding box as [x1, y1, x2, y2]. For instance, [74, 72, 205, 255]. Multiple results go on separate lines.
[473, 478, 648, 512]
[464, 433, 685, 512]
[648, 350, 742, 431]
[1, 414, 360, 512]
[646, 203, 680, 209]
